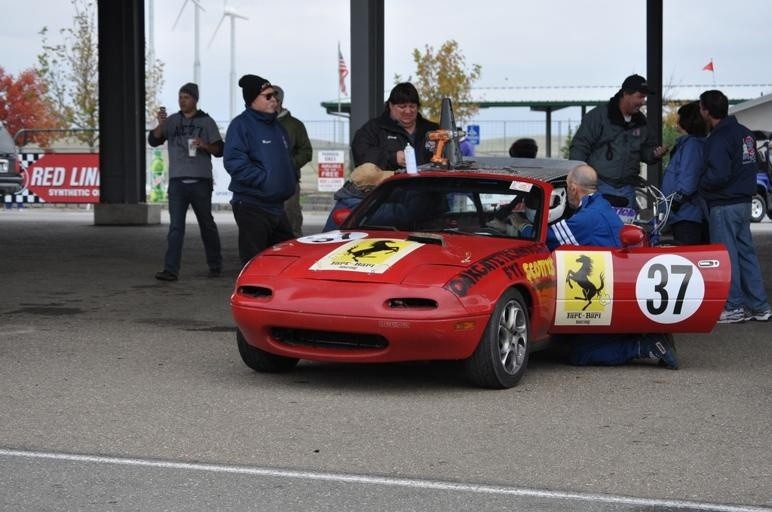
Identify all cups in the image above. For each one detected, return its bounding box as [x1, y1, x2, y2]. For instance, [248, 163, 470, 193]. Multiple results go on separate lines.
[186, 137, 199, 157]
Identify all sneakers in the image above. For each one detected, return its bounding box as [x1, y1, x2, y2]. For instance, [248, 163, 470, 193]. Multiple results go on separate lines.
[155, 270, 177, 281]
[744, 307, 772, 320]
[645, 332, 679, 369]
[717, 307, 745, 323]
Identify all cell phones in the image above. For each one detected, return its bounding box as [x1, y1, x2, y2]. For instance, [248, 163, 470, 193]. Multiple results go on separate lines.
[160, 107, 165, 113]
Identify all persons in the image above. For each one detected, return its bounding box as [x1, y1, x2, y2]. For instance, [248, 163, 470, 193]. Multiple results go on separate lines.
[223, 73, 296, 266]
[656, 100, 709, 246]
[320, 162, 441, 232]
[698, 89, 772, 324]
[485, 164, 679, 372]
[147, 82, 224, 282]
[568, 73, 672, 209]
[271, 85, 312, 237]
[343, 82, 440, 181]
[456, 127, 475, 157]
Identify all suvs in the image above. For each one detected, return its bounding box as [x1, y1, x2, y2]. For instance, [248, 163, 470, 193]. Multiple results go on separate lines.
[0, 121, 22, 195]
[751, 129, 772, 221]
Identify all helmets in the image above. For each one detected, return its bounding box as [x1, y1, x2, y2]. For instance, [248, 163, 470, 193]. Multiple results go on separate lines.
[524, 187, 567, 224]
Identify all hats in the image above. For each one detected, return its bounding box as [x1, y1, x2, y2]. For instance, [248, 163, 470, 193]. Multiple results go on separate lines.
[180, 83, 198, 98]
[239, 74, 271, 104]
[622, 74, 654, 94]
[351, 162, 395, 186]
[272, 86, 283, 101]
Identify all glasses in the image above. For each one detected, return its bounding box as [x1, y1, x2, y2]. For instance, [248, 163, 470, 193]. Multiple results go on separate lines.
[260, 90, 278, 100]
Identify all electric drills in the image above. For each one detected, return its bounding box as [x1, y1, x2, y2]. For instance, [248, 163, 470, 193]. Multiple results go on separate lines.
[425, 129, 472, 170]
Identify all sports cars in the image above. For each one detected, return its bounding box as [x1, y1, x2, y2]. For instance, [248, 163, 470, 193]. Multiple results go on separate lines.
[228, 159, 732, 388]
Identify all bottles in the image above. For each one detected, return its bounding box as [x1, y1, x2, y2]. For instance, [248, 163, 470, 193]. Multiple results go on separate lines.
[403, 143, 418, 176]
[147, 148, 168, 204]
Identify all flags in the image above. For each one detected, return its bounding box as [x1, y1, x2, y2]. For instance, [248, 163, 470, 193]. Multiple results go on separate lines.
[702, 61, 715, 72]
[336, 42, 348, 97]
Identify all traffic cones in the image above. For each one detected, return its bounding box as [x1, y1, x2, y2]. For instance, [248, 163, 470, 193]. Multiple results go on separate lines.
[436, 97, 468, 170]
